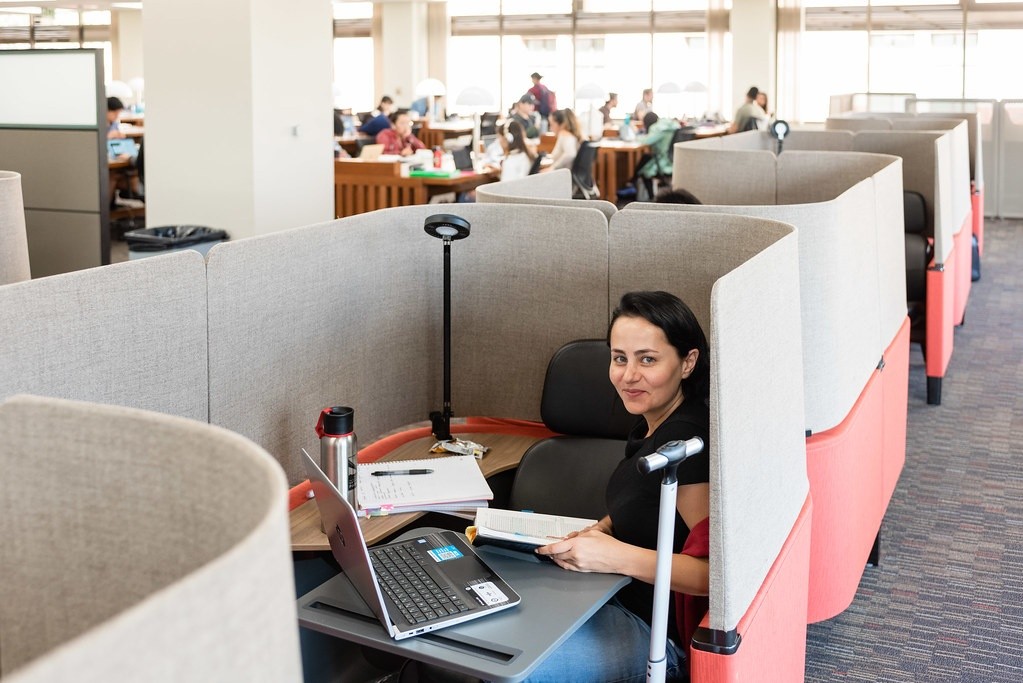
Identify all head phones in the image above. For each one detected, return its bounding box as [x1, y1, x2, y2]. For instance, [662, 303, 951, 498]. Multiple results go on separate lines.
[503, 118, 515, 145]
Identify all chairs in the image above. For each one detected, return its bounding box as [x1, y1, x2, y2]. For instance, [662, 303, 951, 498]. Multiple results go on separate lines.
[903, 190, 929, 366]
[510, 340, 641, 522]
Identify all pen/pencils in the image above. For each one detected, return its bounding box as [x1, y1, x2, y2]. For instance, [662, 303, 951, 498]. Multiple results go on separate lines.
[371, 469, 434, 476]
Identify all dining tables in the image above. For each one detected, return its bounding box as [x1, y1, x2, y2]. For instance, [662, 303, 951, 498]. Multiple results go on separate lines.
[680, 125, 731, 140]
[415, 119, 477, 155]
[407, 168, 474, 204]
[590, 140, 653, 202]
[109, 159, 143, 234]
[600, 121, 650, 140]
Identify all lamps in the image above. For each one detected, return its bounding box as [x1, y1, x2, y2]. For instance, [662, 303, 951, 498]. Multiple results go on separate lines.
[424, 210, 470, 439]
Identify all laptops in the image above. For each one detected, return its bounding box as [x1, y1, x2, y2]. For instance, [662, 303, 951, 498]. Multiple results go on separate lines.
[108, 138, 140, 160]
[359, 145, 387, 159]
[452, 149, 485, 172]
[300, 447, 520, 641]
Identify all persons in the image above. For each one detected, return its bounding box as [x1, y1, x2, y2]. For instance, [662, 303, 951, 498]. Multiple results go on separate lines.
[655, 187, 702, 205]
[726, 87, 770, 134]
[634, 88, 653, 121]
[375, 112, 426, 156]
[616, 112, 681, 202]
[599, 92, 618, 124]
[104, 95, 145, 205]
[477, 291, 710, 683]
[457, 72, 582, 203]
[357, 96, 394, 136]
[333, 116, 352, 159]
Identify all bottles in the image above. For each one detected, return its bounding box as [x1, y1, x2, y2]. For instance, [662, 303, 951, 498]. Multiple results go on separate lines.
[432, 145, 457, 173]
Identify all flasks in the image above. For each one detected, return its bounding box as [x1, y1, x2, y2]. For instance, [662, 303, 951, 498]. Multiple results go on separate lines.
[320, 405, 358, 534]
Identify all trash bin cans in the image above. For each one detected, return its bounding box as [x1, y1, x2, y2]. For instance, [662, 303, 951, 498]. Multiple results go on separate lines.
[123, 225, 226, 261]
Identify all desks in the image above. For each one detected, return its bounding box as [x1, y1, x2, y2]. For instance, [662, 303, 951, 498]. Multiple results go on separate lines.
[297, 527, 632, 683]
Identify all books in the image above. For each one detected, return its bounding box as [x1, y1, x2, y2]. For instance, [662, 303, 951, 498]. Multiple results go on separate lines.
[356, 453, 494, 520]
[473, 508, 600, 552]
